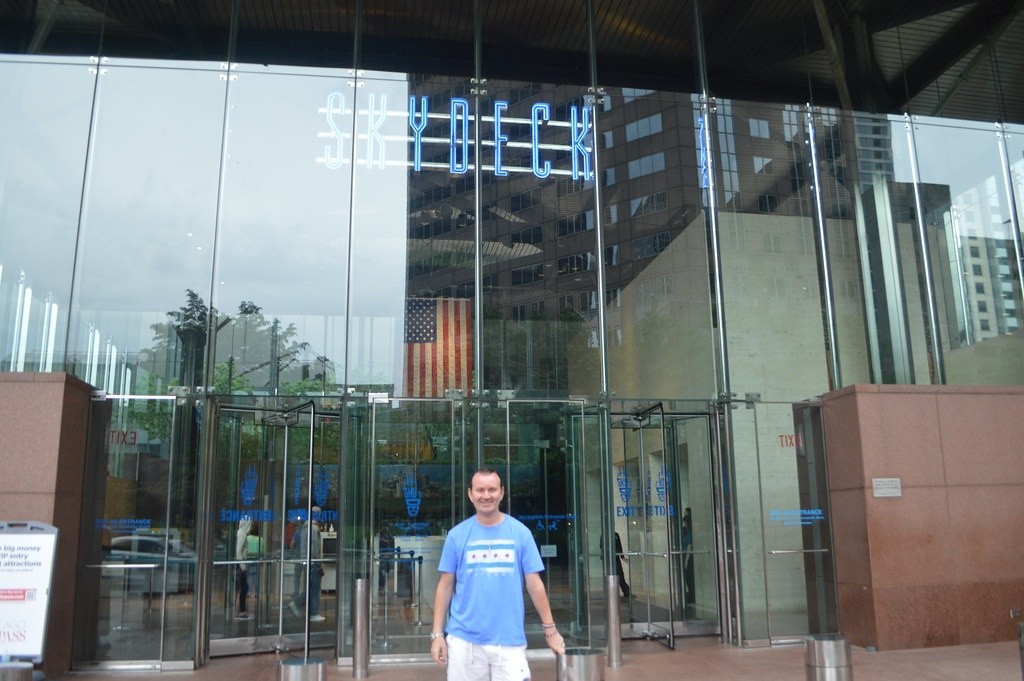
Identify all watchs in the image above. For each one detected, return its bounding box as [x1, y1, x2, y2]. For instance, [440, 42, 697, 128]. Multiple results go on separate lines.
[430, 632, 445, 642]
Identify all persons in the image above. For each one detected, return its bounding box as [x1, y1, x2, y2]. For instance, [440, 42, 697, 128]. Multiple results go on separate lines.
[282, 506, 327, 623]
[430, 468, 567, 681]
[379, 518, 394, 594]
[600, 532, 636, 599]
[234, 514, 254, 619]
[681, 507, 695, 603]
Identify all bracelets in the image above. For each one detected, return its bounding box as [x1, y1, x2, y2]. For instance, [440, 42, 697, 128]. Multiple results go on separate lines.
[540, 622, 558, 637]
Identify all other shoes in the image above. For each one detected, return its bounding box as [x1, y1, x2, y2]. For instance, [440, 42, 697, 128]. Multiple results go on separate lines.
[631, 594, 636, 599]
[235, 613, 254, 620]
[288, 602, 300, 618]
[310, 615, 325, 622]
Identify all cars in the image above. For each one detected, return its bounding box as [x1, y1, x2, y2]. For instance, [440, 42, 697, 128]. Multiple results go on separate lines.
[111, 536, 199, 593]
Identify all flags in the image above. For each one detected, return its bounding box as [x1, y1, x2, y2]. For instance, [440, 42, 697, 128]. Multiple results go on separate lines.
[406, 295, 472, 398]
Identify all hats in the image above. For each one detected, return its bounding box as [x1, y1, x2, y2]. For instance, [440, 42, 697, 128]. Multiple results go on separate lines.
[312, 507, 321, 512]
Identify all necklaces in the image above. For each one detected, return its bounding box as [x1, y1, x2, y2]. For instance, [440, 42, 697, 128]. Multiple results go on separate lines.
[476, 513, 500, 555]
